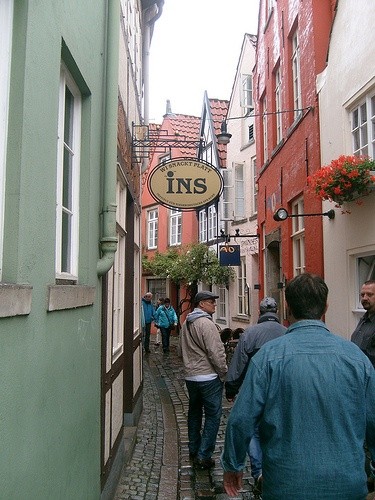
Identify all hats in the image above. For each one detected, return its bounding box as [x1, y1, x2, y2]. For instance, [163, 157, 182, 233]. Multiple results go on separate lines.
[194, 290, 219, 301]
[259, 296, 278, 313]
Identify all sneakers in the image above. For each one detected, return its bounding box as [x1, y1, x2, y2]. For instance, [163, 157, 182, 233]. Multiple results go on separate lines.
[254, 473, 263, 494]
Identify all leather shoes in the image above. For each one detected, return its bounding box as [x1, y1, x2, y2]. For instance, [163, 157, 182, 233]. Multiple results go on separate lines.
[199, 459, 215, 471]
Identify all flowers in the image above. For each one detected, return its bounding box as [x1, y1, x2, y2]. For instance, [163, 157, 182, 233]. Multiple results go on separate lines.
[302, 154, 375, 216]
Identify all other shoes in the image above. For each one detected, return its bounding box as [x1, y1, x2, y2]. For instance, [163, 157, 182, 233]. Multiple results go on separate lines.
[145, 349, 151, 352]
[165, 352, 168, 356]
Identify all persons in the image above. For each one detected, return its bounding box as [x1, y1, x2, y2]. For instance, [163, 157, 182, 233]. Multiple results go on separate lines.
[154, 298, 178, 355]
[179, 291, 228, 468]
[224, 296, 289, 497]
[142, 292, 155, 353]
[154, 297, 165, 346]
[220, 273, 375, 500]
[350, 280, 375, 365]
